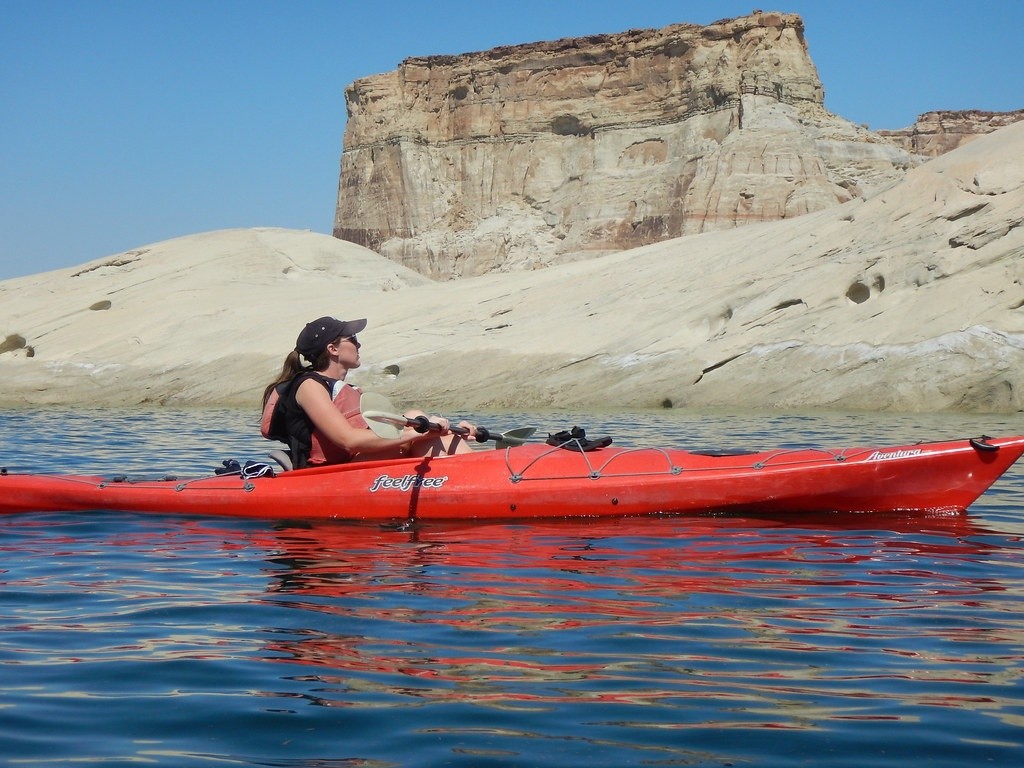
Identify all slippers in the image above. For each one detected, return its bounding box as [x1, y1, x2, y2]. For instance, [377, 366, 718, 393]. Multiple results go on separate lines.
[546, 426, 612, 452]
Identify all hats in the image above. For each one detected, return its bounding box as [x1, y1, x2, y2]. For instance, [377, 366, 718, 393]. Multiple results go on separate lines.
[296, 317, 367, 362]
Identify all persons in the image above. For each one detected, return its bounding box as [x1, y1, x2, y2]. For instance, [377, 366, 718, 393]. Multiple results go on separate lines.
[256, 315, 480, 467]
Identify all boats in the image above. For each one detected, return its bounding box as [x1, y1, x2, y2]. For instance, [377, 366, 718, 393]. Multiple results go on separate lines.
[1, 410, 1024, 529]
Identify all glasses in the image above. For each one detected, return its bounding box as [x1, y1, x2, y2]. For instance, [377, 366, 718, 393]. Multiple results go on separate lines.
[331, 335, 358, 346]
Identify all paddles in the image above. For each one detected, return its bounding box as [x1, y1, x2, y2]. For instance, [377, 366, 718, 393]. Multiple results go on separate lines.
[359, 390, 538, 451]
